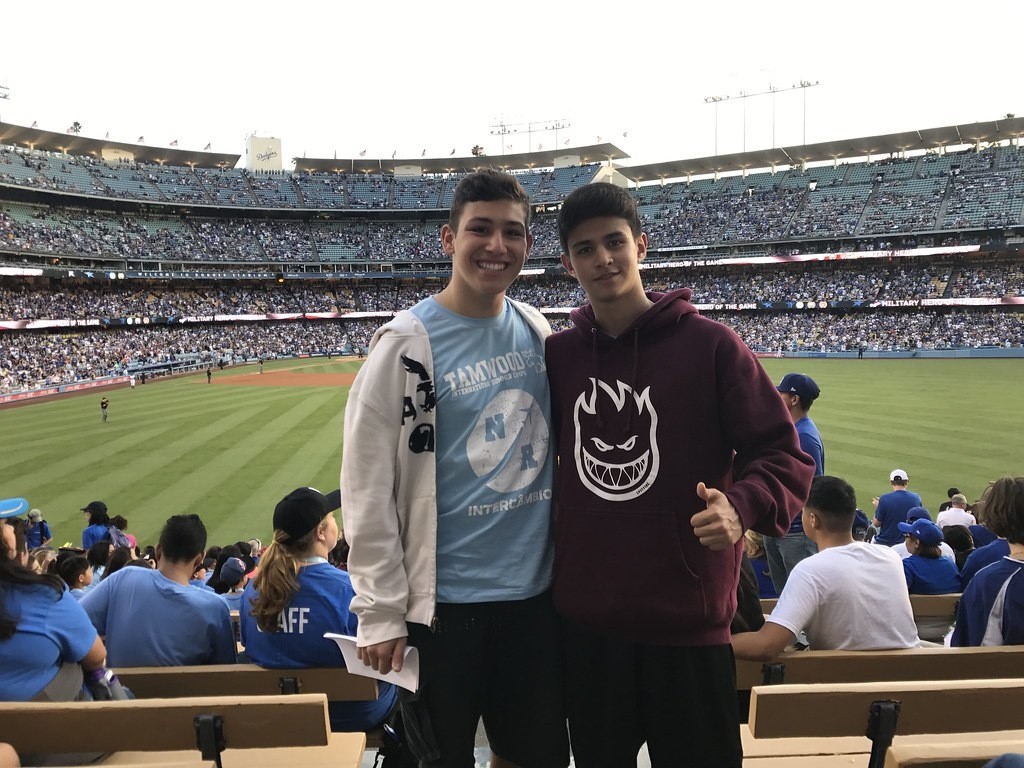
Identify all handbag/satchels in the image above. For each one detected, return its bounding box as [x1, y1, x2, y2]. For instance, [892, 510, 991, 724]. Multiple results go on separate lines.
[106, 525, 132, 549]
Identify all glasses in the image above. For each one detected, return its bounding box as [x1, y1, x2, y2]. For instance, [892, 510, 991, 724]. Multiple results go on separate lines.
[902, 534, 910, 540]
[58, 546, 86, 554]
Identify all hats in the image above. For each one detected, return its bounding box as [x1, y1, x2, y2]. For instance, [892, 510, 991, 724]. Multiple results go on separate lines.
[897, 518, 944, 544]
[952, 493, 967, 503]
[193, 558, 214, 571]
[125, 534, 136, 548]
[906, 506, 932, 523]
[219, 557, 247, 582]
[246, 538, 261, 557]
[273, 486, 341, 539]
[775, 372, 819, 397]
[889, 469, 909, 481]
[80, 501, 107, 512]
[235, 541, 251, 556]
[29, 508, 42, 522]
[0, 498, 29, 518]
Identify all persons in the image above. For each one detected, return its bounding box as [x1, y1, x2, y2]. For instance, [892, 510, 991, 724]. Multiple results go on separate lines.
[732, 371, 1023, 721]
[545, 184, 817, 768]
[340, 171, 574, 768]
[101, 396, 109, 422]
[0, 136, 1024, 386]
[0, 487, 400, 768]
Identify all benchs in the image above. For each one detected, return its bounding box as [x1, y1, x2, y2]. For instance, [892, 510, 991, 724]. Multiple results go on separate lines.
[0, 608, 384, 768]
[734, 592, 1024, 768]
